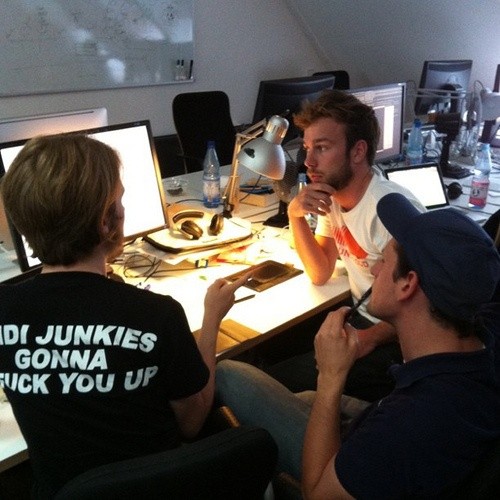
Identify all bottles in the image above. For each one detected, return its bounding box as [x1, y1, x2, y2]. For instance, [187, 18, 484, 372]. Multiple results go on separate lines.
[468, 143, 491, 207]
[406, 118, 423, 164]
[291, 174, 312, 248]
[204, 139, 221, 208]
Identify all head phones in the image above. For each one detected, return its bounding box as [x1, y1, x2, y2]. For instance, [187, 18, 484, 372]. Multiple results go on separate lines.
[171, 208, 224, 239]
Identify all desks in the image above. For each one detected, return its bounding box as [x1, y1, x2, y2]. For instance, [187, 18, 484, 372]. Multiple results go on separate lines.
[0, 146, 500, 474]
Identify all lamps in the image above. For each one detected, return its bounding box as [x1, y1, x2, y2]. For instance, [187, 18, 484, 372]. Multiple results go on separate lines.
[223, 105, 293, 219]
[405, 80, 500, 122]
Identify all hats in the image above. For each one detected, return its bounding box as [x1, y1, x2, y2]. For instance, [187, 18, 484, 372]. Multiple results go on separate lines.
[376, 193, 500, 320]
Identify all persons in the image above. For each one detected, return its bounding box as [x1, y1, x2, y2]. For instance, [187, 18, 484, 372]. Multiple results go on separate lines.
[265, 89, 430, 403]
[215, 194, 500, 500]
[0, 135, 256, 500]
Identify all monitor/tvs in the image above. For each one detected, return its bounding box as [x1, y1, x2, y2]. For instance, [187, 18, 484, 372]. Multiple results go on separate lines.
[338, 80, 406, 165]
[0, 107, 108, 144]
[0, 120, 169, 273]
[250, 73, 335, 151]
[414, 58, 473, 118]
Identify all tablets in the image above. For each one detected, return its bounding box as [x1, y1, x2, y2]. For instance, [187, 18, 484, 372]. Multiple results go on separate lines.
[383, 161, 450, 210]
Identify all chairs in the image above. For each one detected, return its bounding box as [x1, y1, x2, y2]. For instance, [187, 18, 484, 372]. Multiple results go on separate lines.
[171, 91, 237, 169]
[53, 427, 278, 500]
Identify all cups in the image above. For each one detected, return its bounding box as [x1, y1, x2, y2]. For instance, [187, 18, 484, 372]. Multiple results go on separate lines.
[163, 178, 187, 195]
